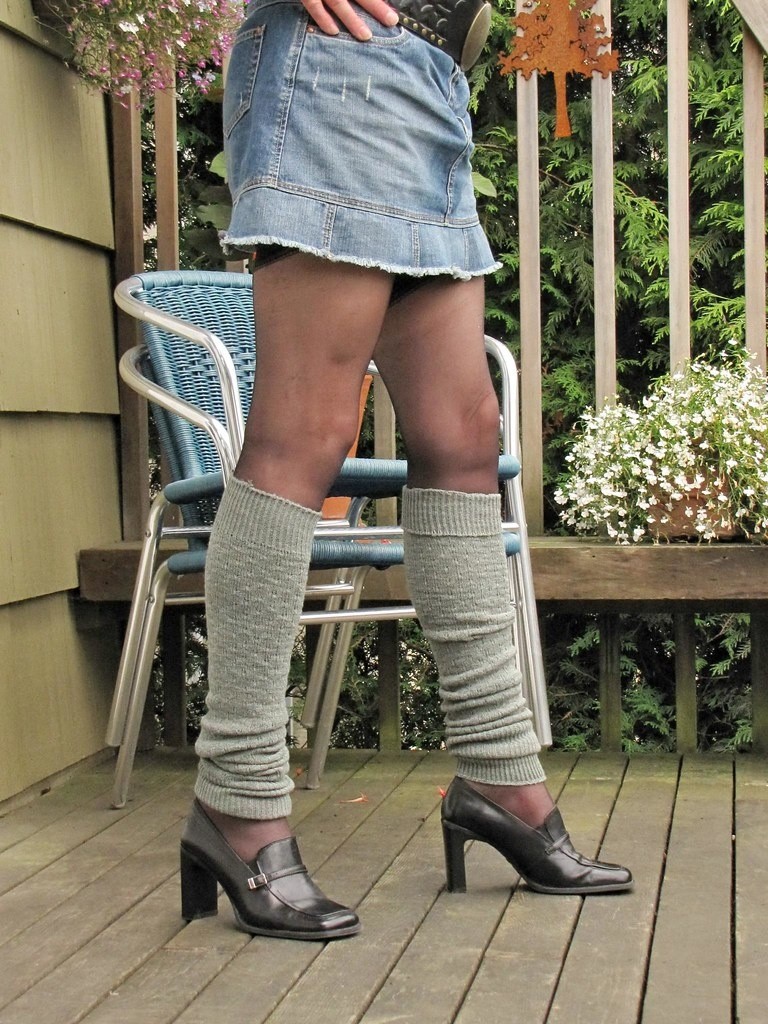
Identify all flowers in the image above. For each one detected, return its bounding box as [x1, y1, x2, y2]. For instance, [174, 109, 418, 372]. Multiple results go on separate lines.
[540, 331, 768, 555]
[45, 0, 249, 116]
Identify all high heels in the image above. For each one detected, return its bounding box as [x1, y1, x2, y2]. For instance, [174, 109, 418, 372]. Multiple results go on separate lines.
[441, 774, 634, 895]
[178, 794, 362, 940]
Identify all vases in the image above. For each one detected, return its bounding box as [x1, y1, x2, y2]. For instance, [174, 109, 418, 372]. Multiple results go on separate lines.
[644, 433, 746, 540]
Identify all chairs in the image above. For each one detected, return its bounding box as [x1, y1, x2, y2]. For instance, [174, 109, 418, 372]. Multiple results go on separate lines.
[106, 269, 554, 813]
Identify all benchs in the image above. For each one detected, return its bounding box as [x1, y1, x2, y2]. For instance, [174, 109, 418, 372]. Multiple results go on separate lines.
[77, 532, 768, 752]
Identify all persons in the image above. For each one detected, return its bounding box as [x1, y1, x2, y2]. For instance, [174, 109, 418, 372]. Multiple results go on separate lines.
[178, 0, 631, 939]
